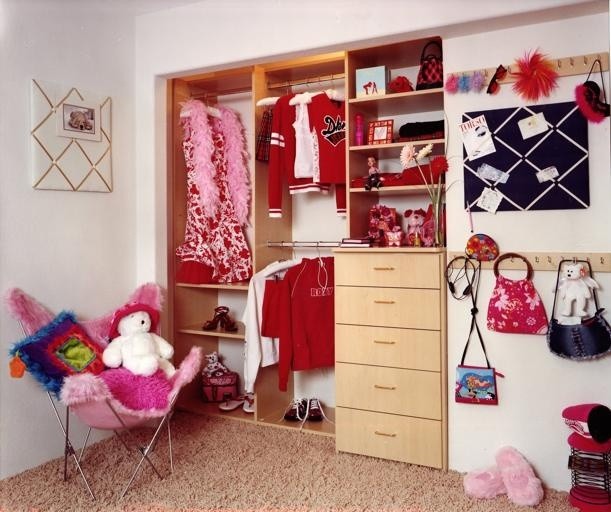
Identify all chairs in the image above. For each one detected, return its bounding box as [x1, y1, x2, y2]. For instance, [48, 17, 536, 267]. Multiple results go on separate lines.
[6, 278, 205, 503]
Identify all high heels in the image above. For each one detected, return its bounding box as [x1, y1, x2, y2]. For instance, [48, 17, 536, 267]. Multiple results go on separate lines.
[202, 306, 225, 331]
[219, 306, 239, 332]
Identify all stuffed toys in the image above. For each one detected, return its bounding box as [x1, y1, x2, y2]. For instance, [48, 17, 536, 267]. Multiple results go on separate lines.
[553, 264, 601, 318]
[104, 301, 176, 382]
[201, 348, 230, 378]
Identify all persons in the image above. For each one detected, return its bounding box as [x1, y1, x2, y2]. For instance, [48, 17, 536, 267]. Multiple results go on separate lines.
[366, 157, 379, 176]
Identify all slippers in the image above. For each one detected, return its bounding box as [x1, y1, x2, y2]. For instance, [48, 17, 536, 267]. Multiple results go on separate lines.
[218, 393, 251, 412]
[242, 393, 255, 413]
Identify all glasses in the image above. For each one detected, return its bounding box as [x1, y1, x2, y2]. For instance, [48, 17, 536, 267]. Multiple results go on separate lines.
[486, 64, 508, 96]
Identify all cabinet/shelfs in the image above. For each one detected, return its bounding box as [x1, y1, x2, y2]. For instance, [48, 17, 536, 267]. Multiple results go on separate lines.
[166, 31, 449, 474]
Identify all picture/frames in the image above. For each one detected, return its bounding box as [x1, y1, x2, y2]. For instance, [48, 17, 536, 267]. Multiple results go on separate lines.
[53, 98, 103, 144]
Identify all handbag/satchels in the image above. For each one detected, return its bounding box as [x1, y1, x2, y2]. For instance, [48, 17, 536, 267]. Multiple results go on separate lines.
[415, 40, 443, 92]
[545, 259, 611, 362]
[485, 252, 549, 335]
[455, 365, 505, 406]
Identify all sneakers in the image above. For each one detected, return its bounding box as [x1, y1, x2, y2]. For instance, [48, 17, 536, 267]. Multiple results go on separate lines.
[283, 399, 307, 421]
[308, 398, 323, 422]
